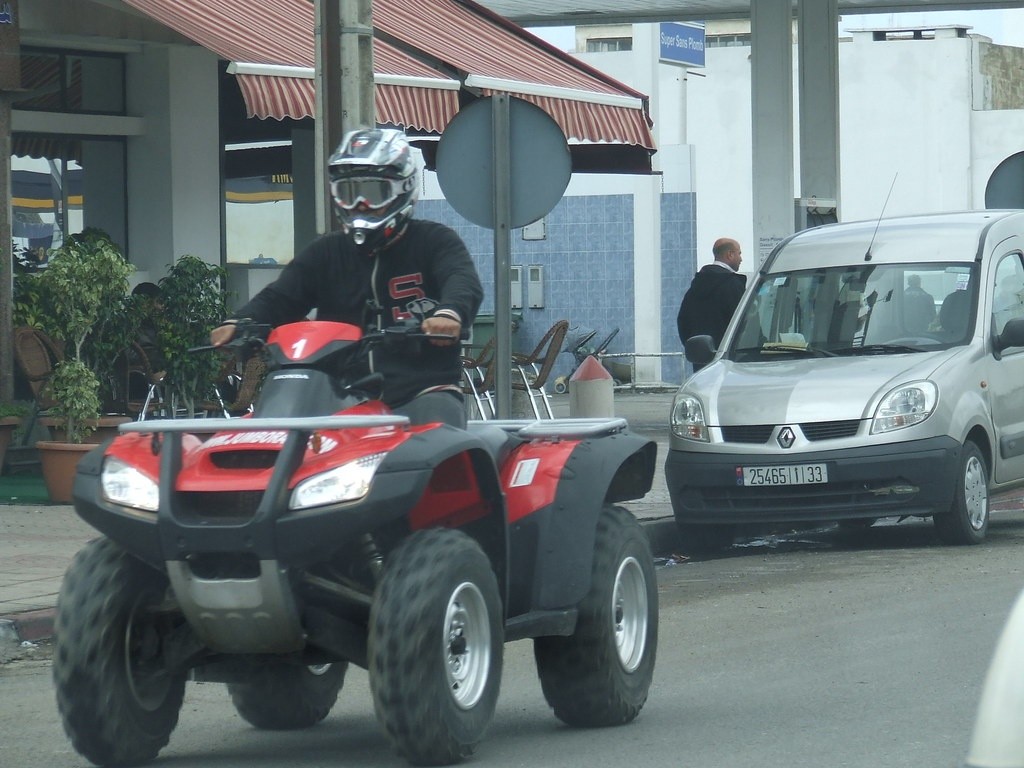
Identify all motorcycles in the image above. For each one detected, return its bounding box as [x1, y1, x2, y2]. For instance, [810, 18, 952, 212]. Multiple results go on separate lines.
[52, 308, 660, 767]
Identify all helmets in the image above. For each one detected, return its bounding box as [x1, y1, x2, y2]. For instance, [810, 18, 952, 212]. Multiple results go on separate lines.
[325, 130, 420, 260]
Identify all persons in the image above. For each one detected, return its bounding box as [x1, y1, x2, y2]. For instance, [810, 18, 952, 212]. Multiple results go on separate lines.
[209, 127, 487, 430]
[676, 236, 765, 374]
[120, 282, 173, 399]
[903, 274, 936, 334]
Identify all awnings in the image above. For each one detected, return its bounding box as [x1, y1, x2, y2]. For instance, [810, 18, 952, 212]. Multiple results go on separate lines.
[8, 53, 86, 167]
[371, 0, 657, 156]
[123, 0, 460, 134]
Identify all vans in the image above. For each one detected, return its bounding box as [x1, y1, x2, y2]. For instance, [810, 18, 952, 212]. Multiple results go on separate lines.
[656, 207, 1022, 546]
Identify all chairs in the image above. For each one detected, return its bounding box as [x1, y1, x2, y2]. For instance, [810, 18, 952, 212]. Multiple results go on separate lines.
[14, 326, 66, 445]
[510, 319, 569, 420]
[937, 289, 972, 341]
[107, 332, 195, 421]
[461, 317, 516, 419]
